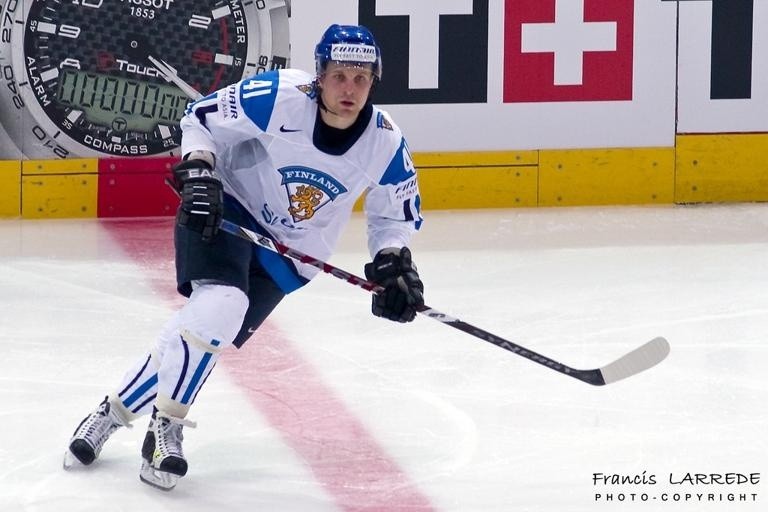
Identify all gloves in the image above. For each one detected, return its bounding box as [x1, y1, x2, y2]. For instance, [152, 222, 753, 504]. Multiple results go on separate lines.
[173, 160, 223, 241]
[365, 247, 424, 322]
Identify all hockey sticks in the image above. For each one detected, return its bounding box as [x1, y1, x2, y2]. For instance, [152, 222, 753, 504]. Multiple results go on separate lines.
[219, 219, 671, 386]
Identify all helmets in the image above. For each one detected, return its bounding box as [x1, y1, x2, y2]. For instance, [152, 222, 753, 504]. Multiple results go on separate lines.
[315, 25, 382, 81]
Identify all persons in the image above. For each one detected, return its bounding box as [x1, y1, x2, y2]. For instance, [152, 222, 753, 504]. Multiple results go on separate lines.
[67, 23, 423, 476]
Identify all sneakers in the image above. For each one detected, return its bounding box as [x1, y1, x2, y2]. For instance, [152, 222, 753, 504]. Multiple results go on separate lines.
[70, 396, 133, 465]
[142, 406, 197, 476]
